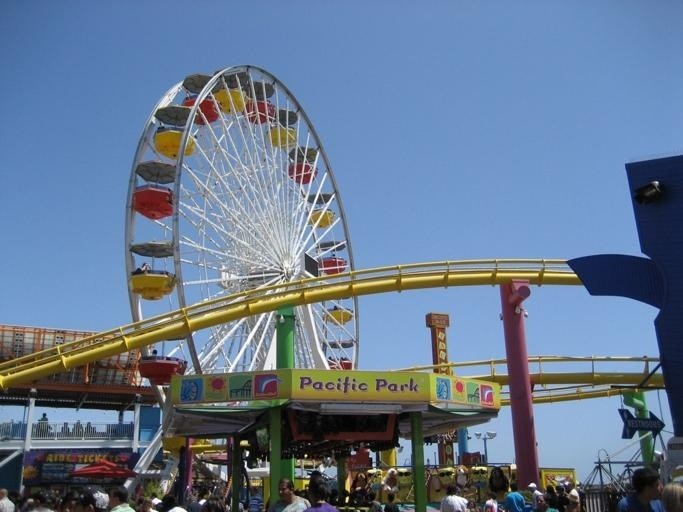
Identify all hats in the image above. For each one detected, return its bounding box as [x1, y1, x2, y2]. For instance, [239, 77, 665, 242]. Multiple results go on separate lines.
[527, 482, 537, 488]
[70, 485, 110, 509]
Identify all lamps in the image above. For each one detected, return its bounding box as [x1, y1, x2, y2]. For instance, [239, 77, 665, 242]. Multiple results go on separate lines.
[633, 179, 665, 206]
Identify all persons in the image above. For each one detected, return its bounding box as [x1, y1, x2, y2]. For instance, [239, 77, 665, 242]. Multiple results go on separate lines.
[152, 478, 337, 511]
[484, 482, 580, 511]
[0, 486, 157, 512]
[615, 465, 664, 511]
[440, 485, 468, 511]
[368, 492, 399, 511]
[660, 481, 682, 511]
